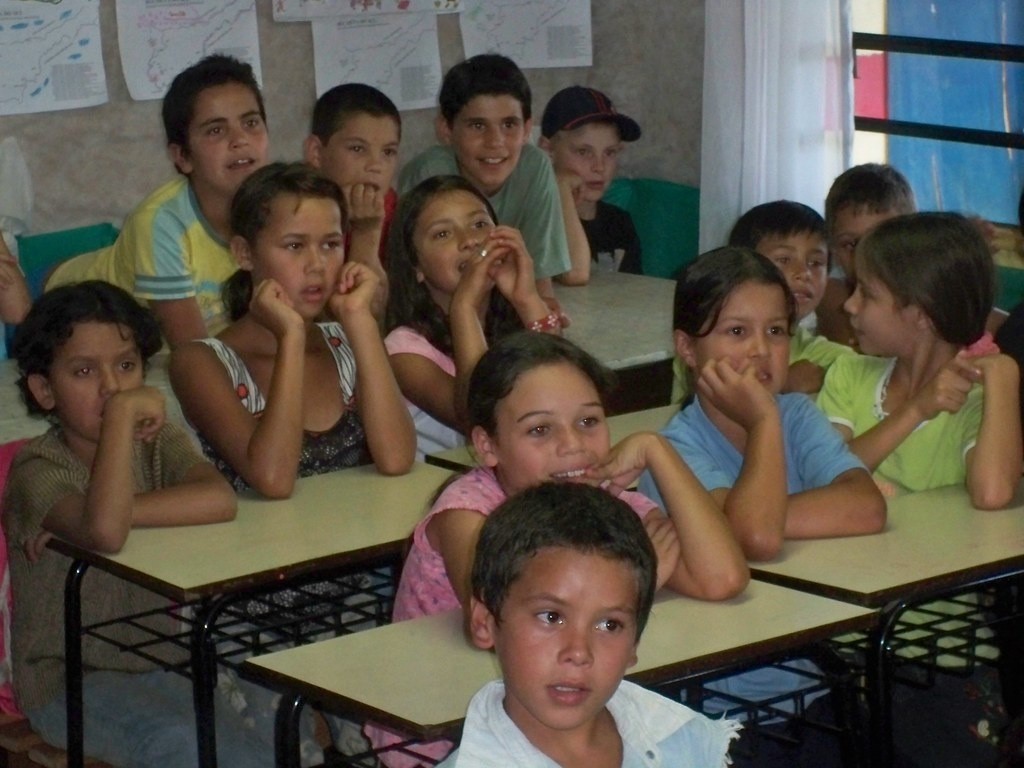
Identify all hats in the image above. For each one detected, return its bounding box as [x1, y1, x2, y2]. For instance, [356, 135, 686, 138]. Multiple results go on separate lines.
[541, 85, 640, 142]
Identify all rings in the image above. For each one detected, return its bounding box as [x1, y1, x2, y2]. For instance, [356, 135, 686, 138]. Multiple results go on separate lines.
[475, 247, 489, 258]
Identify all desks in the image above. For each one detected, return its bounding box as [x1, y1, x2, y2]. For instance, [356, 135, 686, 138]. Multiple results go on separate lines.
[0, 268, 678, 451]
[235, 484, 882, 766]
[421, 400, 678, 495]
[46, 403, 464, 767]
[748, 479, 1023, 766]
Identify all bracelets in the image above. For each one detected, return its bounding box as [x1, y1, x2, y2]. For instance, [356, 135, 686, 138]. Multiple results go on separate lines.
[522, 308, 560, 334]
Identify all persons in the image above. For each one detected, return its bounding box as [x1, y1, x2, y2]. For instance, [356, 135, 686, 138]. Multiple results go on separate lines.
[361, 331, 750, 768]
[633, 244, 1011, 768]
[817, 210, 1024, 753]
[302, 80, 402, 337]
[393, 54, 573, 329]
[379, 173, 572, 466]
[536, 86, 646, 286]
[167, 164, 418, 768]
[0, 226, 32, 365]
[0, 282, 277, 768]
[434, 480, 746, 768]
[5, 54, 270, 363]
[812, 162, 1011, 355]
[670, 201, 859, 402]
[992, 185, 1024, 439]
